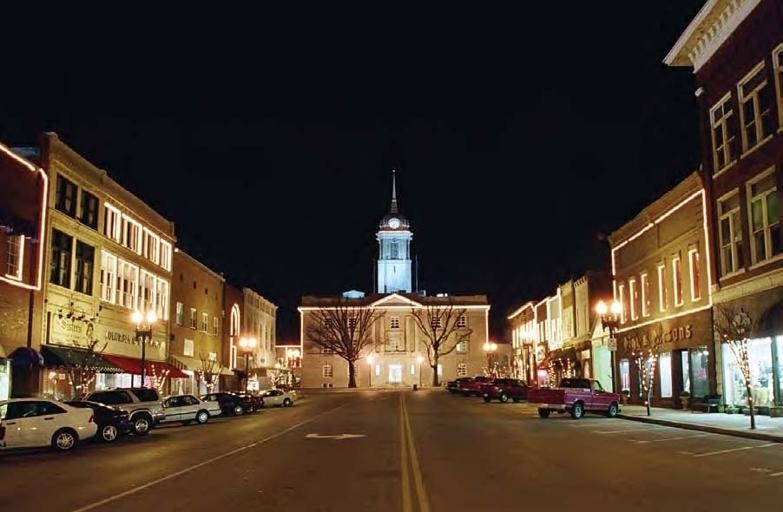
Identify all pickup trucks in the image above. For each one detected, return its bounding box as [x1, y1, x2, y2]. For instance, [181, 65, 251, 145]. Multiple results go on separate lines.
[526, 377, 623, 420]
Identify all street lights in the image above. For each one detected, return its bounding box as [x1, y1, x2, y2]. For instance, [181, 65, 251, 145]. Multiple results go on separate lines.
[483, 341, 497, 378]
[522, 329, 537, 387]
[595, 299, 624, 413]
[238, 334, 257, 391]
[286, 348, 302, 386]
[131, 309, 158, 390]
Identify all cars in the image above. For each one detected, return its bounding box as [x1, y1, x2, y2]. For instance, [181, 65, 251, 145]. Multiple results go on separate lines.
[448, 374, 537, 404]
